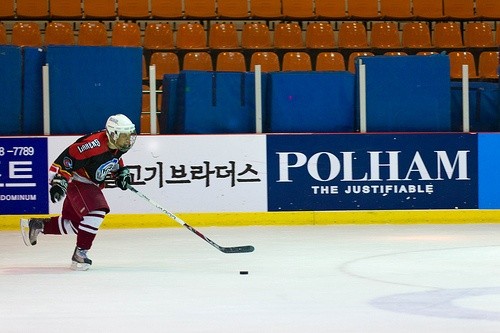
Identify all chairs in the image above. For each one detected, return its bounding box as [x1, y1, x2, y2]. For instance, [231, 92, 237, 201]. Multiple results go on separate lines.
[0, 0, 500, 134]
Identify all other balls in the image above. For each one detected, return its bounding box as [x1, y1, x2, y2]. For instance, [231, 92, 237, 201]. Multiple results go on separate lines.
[239, 270, 249, 275]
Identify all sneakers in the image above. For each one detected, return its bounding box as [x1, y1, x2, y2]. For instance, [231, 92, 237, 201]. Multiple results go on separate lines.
[69, 247, 93, 272]
[20, 218, 44, 248]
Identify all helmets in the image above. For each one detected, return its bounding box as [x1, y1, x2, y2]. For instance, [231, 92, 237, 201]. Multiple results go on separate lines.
[106, 114, 137, 152]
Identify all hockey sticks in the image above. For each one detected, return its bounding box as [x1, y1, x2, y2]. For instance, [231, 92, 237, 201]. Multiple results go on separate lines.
[112, 172, 256, 253]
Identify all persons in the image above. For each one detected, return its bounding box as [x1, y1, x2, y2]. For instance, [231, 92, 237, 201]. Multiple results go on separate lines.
[19, 114, 139, 271]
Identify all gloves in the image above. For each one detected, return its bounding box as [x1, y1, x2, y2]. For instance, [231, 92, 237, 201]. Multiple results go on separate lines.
[112, 166, 130, 191]
[50, 174, 66, 204]
